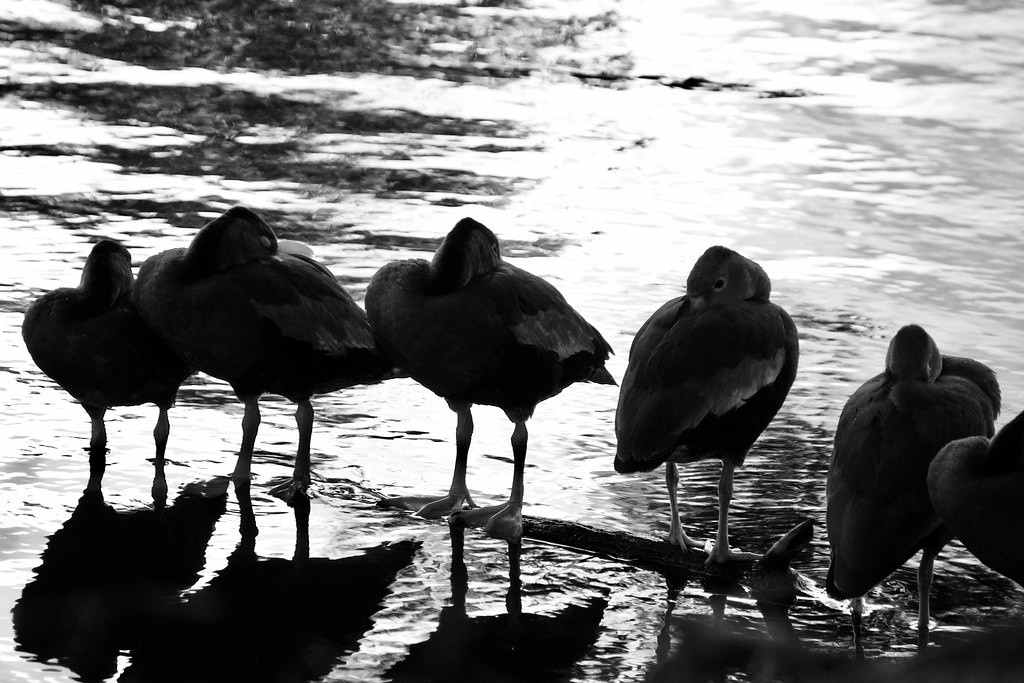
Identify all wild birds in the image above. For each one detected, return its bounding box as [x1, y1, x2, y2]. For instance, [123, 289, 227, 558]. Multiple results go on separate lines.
[926, 408, 1024, 592]
[612, 246, 800, 567]
[21, 240, 198, 463]
[365, 218, 620, 540]
[134, 206, 400, 505]
[827, 326, 1000, 659]
